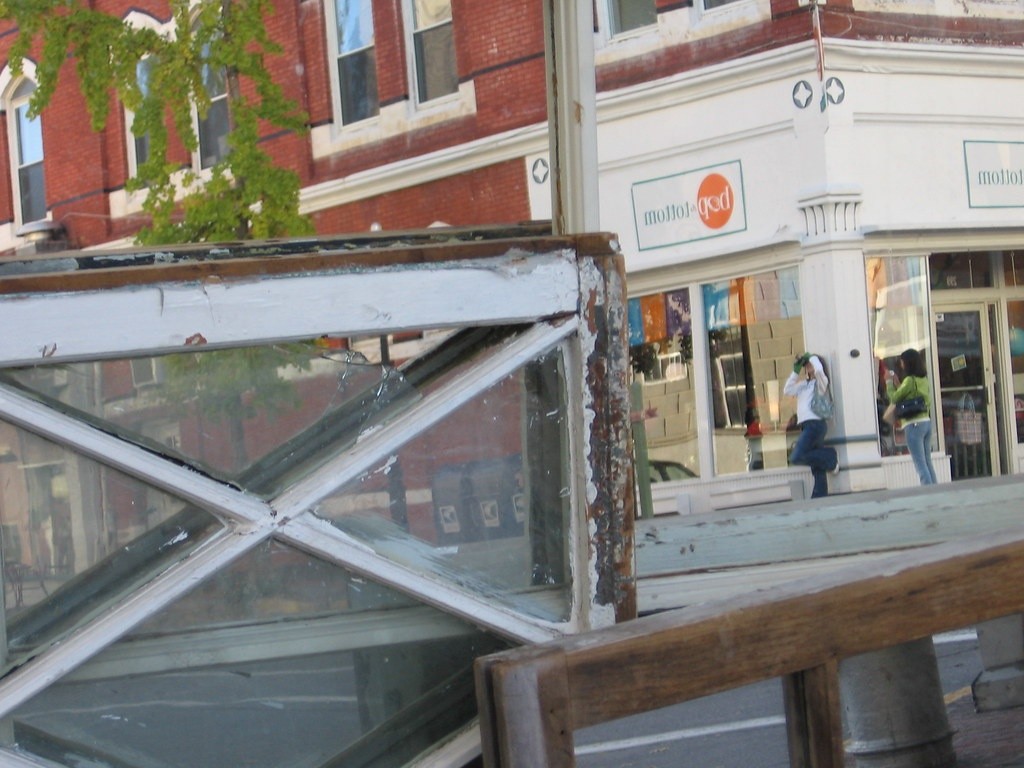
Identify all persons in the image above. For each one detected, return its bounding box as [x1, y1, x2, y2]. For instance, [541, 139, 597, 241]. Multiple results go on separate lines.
[783, 351, 840, 500]
[884, 348, 937, 484]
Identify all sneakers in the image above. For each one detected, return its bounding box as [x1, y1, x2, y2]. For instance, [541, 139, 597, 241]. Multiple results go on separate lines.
[830, 450, 839, 475]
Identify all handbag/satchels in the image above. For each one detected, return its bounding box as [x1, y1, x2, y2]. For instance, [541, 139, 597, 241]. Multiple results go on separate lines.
[810, 379, 834, 420]
[953, 392, 981, 445]
[895, 376, 927, 418]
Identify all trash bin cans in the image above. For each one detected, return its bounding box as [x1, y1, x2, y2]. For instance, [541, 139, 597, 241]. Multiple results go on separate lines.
[427, 452, 525, 546]
